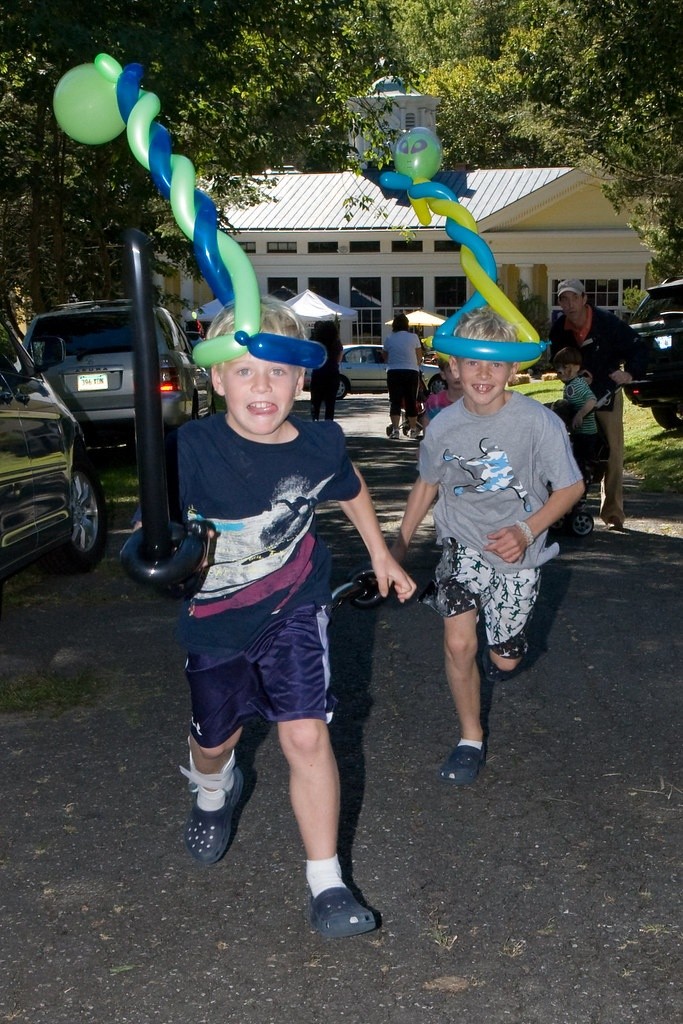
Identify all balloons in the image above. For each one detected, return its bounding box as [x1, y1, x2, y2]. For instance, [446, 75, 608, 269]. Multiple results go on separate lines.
[55, 53, 327, 369]
[379, 127, 546, 371]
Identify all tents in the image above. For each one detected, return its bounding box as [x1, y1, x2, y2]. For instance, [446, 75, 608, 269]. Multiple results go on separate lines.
[185, 298, 224, 331]
[271, 286, 297, 301]
[331, 287, 381, 307]
[285, 289, 362, 363]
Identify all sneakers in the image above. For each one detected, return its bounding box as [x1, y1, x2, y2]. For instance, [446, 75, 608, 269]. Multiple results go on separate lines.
[389, 429, 399, 439]
[409, 426, 421, 439]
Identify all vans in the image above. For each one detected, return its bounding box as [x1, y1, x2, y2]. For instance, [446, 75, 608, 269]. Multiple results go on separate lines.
[0, 305, 109, 583]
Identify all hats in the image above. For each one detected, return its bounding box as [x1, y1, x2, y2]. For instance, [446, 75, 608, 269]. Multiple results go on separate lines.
[557, 278, 586, 301]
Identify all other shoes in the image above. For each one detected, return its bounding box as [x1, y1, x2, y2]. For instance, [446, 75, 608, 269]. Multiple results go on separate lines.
[607, 516, 623, 531]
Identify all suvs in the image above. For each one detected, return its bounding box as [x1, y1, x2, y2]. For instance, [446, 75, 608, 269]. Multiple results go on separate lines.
[21, 297, 216, 472]
[623, 276, 683, 430]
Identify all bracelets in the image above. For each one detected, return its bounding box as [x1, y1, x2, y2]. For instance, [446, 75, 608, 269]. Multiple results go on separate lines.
[516, 520, 535, 546]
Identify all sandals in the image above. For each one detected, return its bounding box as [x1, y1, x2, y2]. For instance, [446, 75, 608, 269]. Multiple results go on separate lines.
[307, 883, 378, 940]
[183, 766, 245, 865]
[483, 652, 505, 683]
[437, 739, 486, 784]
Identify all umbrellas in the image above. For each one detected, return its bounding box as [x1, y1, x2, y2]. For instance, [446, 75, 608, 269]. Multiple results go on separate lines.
[386, 308, 450, 327]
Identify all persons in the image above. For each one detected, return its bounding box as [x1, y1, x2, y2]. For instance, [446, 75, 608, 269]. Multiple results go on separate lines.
[390, 305, 585, 785]
[134, 292, 418, 936]
[424, 278, 647, 554]
[383, 313, 423, 440]
[309, 321, 343, 421]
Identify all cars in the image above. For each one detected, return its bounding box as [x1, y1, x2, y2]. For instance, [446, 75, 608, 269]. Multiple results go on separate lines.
[302, 342, 448, 400]
